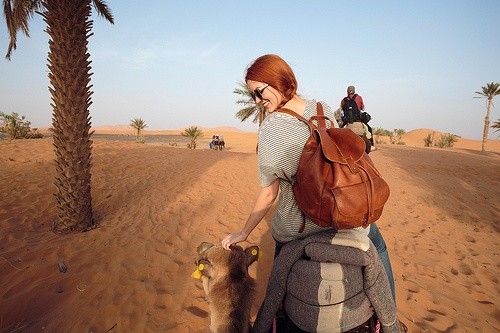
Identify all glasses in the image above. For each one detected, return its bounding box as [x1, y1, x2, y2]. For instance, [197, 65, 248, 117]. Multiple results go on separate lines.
[249, 83, 270, 102]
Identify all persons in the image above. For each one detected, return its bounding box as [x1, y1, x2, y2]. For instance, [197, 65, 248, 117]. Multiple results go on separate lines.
[221, 54, 398, 310]
[210, 134, 225, 150]
[339, 86, 377, 151]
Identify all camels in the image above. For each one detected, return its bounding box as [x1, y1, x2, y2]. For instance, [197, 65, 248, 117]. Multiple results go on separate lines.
[190, 241, 261, 333]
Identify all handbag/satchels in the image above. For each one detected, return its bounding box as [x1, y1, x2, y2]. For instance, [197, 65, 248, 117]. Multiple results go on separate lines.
[362, 111, 371, 122]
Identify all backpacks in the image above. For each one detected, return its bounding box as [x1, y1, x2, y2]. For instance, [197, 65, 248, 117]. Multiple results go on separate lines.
[342, 94, 361, 119]
[275, 102, 390, 232]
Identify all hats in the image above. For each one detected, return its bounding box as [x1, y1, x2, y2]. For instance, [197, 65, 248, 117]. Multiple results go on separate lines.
[347, 86, 355, 93]
